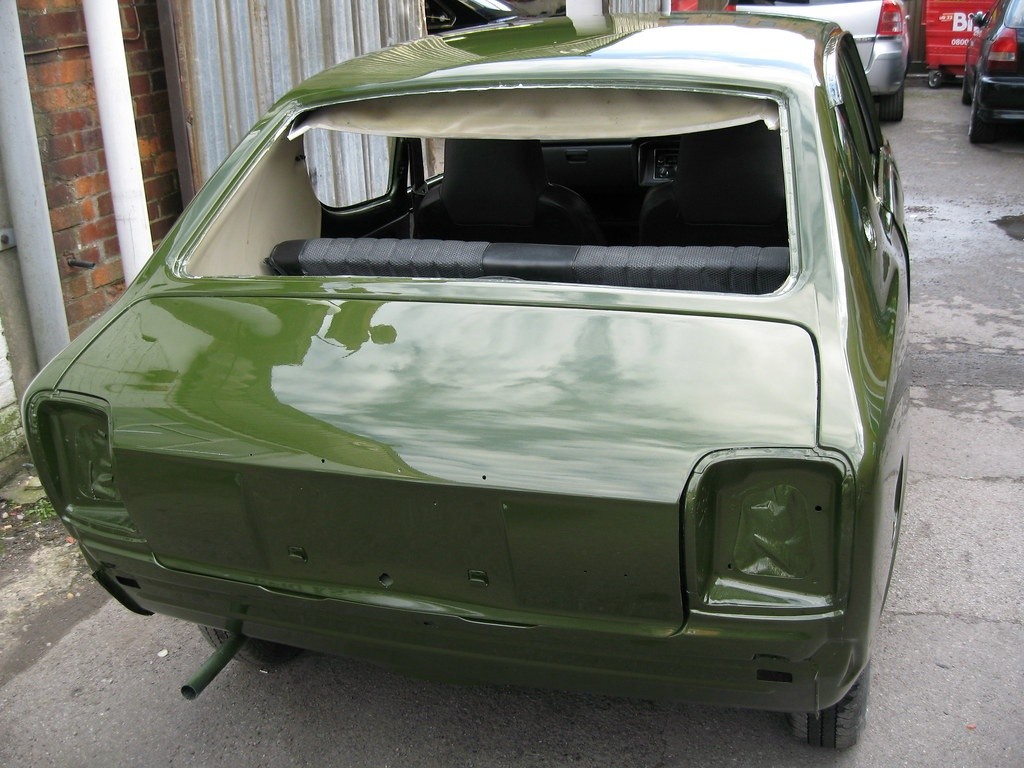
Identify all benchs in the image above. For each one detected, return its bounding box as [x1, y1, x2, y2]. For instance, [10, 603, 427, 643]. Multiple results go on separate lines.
[266, 240, 790, 294]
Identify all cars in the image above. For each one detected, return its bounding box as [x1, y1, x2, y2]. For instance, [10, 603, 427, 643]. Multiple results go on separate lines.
[921, 1, 998, 89]
[21, 11, 913, 750]
[425, 0, 565, 34]
[962, 0, 1024, 143]
[721, 0, 910, 120]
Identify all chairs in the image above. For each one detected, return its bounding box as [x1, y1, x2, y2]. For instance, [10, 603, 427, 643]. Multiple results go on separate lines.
[417, 138, 606, 245]
[638, 120, 788, 248]
